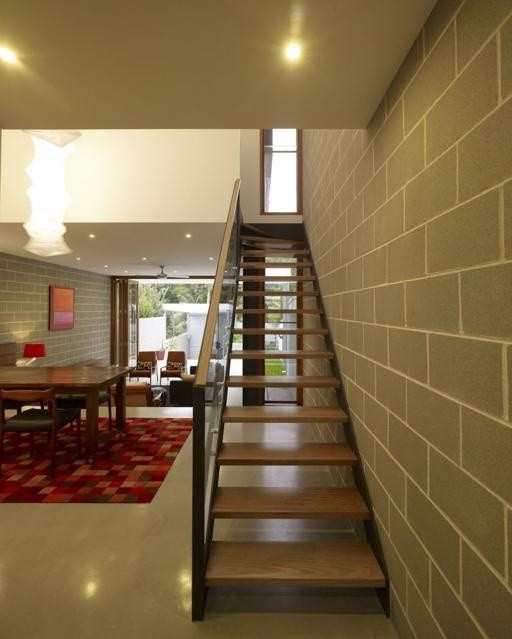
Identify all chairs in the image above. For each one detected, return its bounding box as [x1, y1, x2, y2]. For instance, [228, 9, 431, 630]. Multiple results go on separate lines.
[107, 381, 168, 407]
[0, 387, 81, 479]
[47, 386, 111, 447]
[129, 351, 187, 388]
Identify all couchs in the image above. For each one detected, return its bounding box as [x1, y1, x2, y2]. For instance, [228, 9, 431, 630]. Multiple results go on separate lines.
[169, 366, 224, 405]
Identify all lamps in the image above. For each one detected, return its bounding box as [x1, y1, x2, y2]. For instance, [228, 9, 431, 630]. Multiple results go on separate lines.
[21, 343, 46, 366]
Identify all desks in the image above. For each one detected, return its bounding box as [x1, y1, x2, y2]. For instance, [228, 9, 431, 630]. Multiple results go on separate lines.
[0, 366, 135, 464]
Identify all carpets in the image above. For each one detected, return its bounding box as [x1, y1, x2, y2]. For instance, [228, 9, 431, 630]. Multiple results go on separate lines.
[0, 417, 193, 504]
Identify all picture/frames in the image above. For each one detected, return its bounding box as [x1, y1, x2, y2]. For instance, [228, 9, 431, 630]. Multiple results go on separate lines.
[48, 285, 75, 330]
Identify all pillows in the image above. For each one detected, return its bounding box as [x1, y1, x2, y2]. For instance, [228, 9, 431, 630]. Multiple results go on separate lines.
[179, 372, 195, 382]
